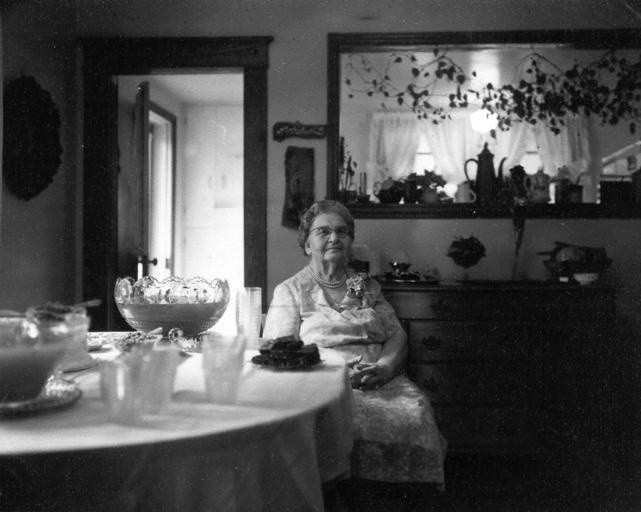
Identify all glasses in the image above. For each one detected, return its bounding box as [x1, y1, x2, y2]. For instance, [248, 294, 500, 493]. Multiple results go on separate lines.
[310, 226, 351, 238]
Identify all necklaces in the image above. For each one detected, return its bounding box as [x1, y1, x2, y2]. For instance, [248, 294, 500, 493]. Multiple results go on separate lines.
[306, 265, 348, 289]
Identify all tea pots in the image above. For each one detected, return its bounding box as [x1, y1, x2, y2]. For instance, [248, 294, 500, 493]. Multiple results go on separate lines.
[522, 168, 557, 203]
[371, 173, 426, 205]
[465, 141, 509, 207]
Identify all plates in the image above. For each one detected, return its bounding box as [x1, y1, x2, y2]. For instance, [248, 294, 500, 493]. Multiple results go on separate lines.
[1, 377, 85, 414]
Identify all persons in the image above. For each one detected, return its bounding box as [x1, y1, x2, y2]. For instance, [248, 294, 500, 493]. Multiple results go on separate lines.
[261, 200, 448, 511]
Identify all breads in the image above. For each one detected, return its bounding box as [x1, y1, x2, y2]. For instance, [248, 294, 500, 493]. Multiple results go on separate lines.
[251, 336, 321, 372]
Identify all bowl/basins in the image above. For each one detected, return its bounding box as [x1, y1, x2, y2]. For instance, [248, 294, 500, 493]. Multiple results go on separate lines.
[0, 315, 76, 404]
[573, 272, 600, 286]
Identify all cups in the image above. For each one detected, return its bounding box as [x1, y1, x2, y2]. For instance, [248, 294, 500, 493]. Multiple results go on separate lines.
[199, 333, 250, 404]
[388, 246, 413, 278]
[112, 344, 179, 427]
[455, 182, 477, 204]
[232, 286, 264, 346]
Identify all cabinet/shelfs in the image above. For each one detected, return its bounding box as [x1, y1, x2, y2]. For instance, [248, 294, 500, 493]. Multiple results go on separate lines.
[380, 286, 621, 456]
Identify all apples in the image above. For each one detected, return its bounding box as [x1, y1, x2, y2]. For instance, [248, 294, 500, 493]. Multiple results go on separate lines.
[555, 244, 585, 262]
